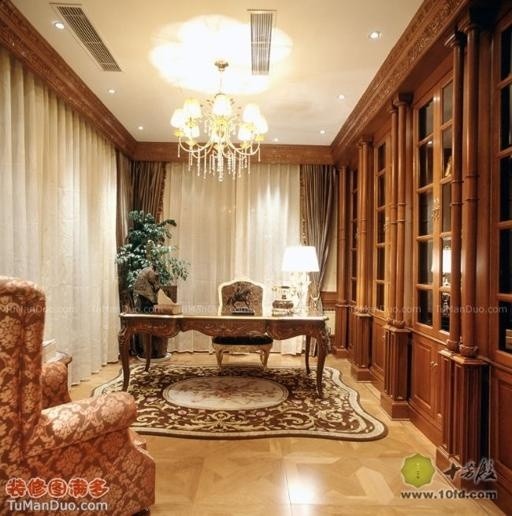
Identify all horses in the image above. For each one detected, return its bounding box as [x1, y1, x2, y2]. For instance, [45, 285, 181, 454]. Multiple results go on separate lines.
[226, 287, 252, 309]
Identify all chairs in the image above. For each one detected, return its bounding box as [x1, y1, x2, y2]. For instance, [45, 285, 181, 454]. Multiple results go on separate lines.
[0, 275, 156, 516]
[211, 279, 273, 373]
[45, 352, 73, 368]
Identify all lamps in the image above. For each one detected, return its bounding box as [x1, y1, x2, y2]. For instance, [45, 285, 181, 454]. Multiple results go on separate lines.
[277, 245, 321, 313]
[430, 247, 452, 317]
[170, 60, 268, 183]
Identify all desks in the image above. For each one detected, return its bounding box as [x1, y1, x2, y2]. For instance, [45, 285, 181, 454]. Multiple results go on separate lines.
[118, 313, 329, 399]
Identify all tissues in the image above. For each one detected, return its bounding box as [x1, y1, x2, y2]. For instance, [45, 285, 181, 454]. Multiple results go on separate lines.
[154, 288, 181, 315]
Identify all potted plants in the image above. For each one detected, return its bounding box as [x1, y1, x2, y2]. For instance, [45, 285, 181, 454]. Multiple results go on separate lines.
[113, 210, 193, 358]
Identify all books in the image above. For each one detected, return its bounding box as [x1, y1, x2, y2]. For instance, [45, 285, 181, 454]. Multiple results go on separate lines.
[153, 305, 178, 315]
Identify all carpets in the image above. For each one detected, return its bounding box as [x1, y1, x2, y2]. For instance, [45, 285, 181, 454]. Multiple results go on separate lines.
[90, 362, 389, 442]
